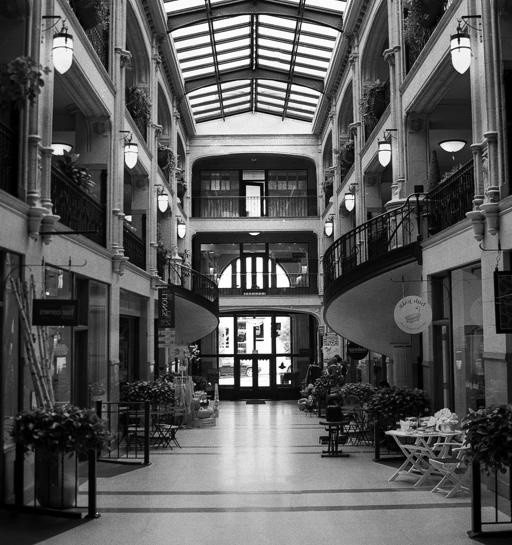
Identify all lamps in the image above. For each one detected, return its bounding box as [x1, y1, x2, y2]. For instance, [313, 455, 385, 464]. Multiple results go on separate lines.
[154, 183, 187, 242]
[439, 140, 467, 155]
[118, 128, 139, 171]
[448, 14, 483, 75]
[51, 143, 72, 158]
[39, 13, 74, 74]
[376, 128, 395, 168]
[323, 182, 356, 237]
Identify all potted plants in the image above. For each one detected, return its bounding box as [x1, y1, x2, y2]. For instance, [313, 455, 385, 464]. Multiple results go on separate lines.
[363, 386, 433, 451]
[8, 399, 114, 509]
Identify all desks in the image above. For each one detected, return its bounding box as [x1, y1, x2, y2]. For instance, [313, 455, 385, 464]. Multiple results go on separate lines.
[384, 429, 463, 488]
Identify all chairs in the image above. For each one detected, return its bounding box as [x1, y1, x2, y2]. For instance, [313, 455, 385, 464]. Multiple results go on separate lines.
[108, 406, 185, 451]
[318, 393, 370, 458]
[429, 413, 484, 498]
